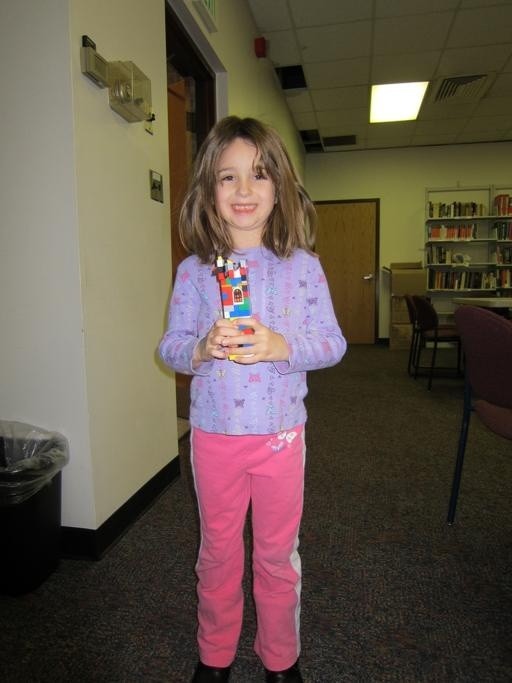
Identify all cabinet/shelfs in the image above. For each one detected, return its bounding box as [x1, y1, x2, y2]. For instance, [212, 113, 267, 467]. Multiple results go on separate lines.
[422, 183, 511, 292]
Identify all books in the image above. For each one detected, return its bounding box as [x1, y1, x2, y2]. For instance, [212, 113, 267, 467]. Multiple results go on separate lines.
[426, 192, 511, 290]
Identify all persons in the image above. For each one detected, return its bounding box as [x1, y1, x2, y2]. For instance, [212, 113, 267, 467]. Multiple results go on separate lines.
[155, 112, 350, 681]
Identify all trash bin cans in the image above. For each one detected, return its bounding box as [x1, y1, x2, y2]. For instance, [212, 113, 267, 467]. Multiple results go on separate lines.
[2, 437, 70, 597]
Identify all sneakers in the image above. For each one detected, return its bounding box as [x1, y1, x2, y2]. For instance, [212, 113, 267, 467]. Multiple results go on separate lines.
[186, 658, 234, 682]
[265, 658, 304, 683]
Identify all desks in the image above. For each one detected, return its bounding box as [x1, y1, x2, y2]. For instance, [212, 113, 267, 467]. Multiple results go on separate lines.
[452, 296, 512, 316]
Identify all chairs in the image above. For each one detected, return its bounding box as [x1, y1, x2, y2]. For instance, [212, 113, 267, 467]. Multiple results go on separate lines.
[445, 303, 512, 528]
[403, 292, 464, 389]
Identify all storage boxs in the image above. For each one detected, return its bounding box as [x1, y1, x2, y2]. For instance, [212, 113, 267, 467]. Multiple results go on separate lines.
[381, 260, 423, 351]
